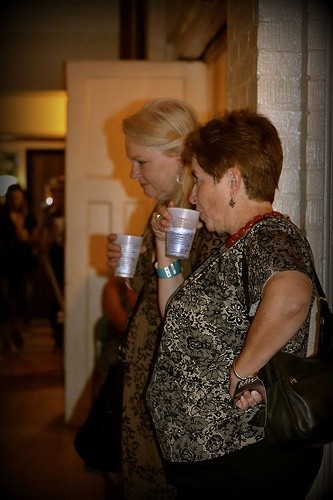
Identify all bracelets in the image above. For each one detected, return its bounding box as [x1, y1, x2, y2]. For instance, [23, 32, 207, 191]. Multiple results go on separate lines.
[232, 356, 261, 381]
[153, 258, 183, 279]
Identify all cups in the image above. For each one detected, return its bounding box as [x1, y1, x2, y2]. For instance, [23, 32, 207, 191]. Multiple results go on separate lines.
[166, 207, 199, 258]
[113, 234, 142, 279]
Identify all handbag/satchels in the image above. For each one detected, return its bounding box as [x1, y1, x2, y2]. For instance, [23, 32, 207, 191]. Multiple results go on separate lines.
[241, 217, 333, 449]
[73, 353, 135, 471]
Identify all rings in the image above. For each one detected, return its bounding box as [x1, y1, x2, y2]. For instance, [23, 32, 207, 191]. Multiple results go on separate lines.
[156, 214, 163, 223]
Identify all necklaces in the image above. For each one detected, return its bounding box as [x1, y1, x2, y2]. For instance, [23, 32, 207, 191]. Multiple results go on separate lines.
[225, 206, 286, 248]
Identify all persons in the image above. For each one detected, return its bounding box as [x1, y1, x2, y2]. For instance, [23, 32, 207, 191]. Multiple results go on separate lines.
[0, 169, 66, 356]
[142, 108, 323, 500]
[106, 98, 233, 500]
[74, 265, 141, 476]
